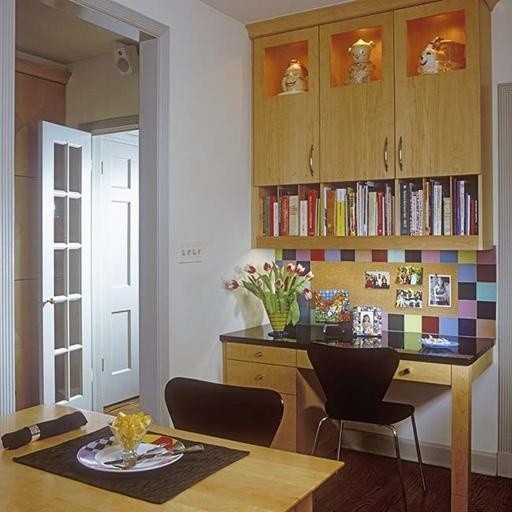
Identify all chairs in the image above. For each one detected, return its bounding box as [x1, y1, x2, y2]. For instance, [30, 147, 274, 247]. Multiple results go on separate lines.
[305, 341, 428, 512]
[160, 378, 283, 451]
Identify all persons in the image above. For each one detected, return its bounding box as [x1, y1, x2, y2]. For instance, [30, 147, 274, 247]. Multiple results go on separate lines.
[433, 276, 449, 305]
[396, 267, 421, 285]
[396, 289, 422, 308]
[365, 272, 390, 290]
[362, 314, 373, 335]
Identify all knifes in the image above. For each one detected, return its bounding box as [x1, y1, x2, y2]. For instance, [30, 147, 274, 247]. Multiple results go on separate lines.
[104, 444, 208, 468]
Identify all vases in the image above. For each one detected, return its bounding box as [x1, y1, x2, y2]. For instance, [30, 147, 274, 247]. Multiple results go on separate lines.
[260, 294, 297, 337]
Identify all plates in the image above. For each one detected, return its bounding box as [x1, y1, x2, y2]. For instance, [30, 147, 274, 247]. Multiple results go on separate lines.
[419, 338, 461, 350]
[76, 435, 185, 473]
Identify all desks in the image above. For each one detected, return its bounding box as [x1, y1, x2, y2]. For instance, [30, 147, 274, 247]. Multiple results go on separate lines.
[220, 321, 495, 512]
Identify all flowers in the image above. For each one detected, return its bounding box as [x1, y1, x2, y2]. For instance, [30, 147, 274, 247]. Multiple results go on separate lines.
[222, 259, 316, 314]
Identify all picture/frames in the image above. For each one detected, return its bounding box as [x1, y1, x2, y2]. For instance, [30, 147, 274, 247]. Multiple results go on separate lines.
[351, 305, 384, 338]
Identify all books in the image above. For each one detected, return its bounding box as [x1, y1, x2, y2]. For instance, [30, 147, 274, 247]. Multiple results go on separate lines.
[259, 179, 479, 238]
